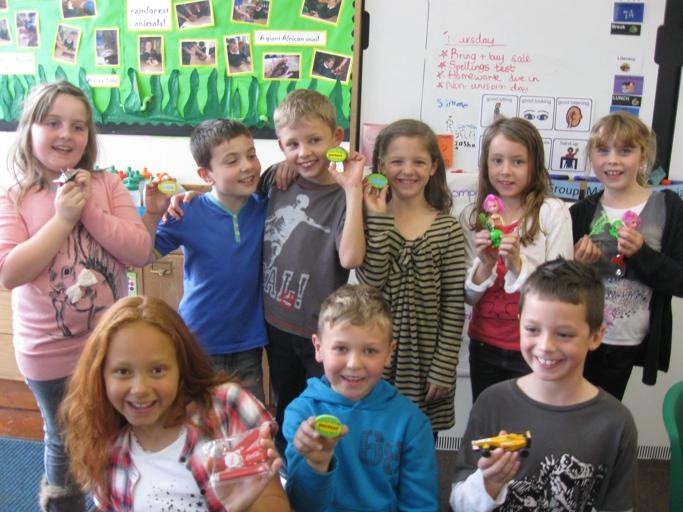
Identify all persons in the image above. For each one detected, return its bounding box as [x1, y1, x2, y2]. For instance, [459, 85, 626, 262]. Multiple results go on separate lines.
[446, 253, 637, 512]
[605, 211, 641, 269]
[459, 117, 574, 406]
[57, 294, 283, 511]
[142, 118, 299, 405]
[570, 113, 683, 402]
[1, 82, 151, 512]
[355, 118, 465, 445]
[161, 88, 366, 490]
[281, 283, 441, 512]
[478, 194, 522, 254]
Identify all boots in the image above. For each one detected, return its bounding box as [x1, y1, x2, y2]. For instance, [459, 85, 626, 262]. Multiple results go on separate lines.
[37, 476, 86, 511]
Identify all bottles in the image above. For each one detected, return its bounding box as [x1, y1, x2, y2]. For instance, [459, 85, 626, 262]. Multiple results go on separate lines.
[109, 165, 153, 208]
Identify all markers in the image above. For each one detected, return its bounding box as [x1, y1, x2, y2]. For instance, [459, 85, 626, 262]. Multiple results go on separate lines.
[660, 179, 683, 185]
[549, 174, 569, 181]
[574, 176, 599, 182]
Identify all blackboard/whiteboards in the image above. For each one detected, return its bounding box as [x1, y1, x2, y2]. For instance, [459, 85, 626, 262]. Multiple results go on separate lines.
[349, 0, 683, 185]
